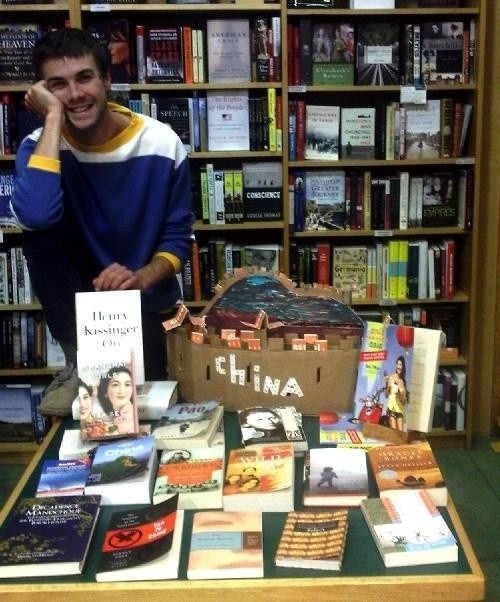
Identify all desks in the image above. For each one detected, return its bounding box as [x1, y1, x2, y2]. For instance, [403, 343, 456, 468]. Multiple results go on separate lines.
[0, 396, 486, 602]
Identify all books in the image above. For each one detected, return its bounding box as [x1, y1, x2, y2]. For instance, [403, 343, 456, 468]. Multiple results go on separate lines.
[0, 401, 460, 581]
[193, 161, 284, 224]
[287, 1, 477, 86]
[288, 167, 473, 230]
[288, 96, 472, 160]
[123, 88, 283, 152]
[173, 233, 286, 299]
[68, 287, 181, 445]
[354, 306, 468, 433]
[289, 239, 454, 301]
[87, 17, 282, 84]
[0, 23, 67, 441]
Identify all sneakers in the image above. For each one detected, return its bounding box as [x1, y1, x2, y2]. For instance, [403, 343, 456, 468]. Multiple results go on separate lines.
[37, 363, 79, 417]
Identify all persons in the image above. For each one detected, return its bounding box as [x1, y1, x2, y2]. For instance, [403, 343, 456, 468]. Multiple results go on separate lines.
[312, 24, 355, 65]
[11, 26, 194, 418]
[422, 24, 463, 73]
[77, 366, 137, 438]
